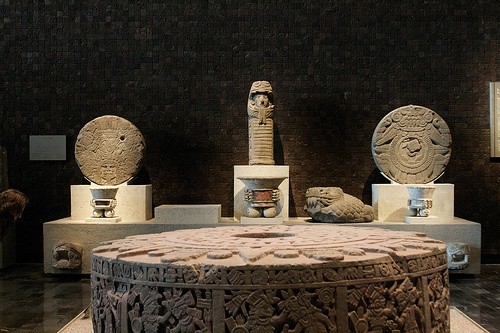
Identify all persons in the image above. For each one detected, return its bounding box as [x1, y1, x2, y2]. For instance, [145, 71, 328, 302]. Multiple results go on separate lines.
[0, 189, 29, 242]
